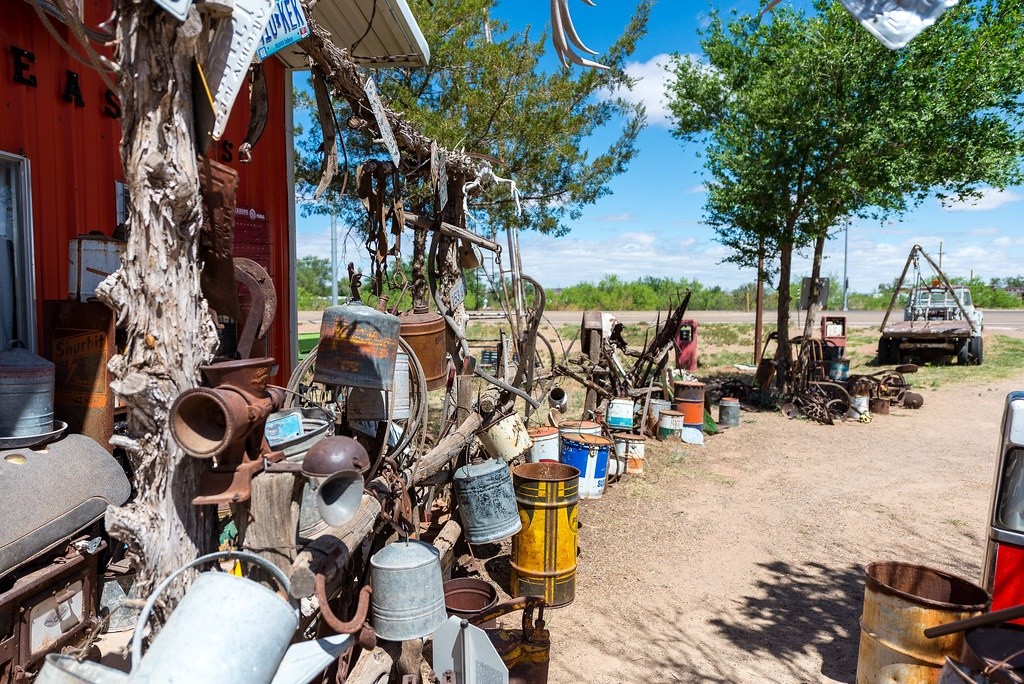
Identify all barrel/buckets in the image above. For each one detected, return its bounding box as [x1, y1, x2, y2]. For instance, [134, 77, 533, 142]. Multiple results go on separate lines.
[937, 648, 1024, 684]
[606, 398, 634, 430]
[658, 404, 685, 438]
[829, 358, 850, 380]
[0, 230, 606, 684]
[850, 397, 869, 419]
[855, 560, 992, 684]
[612, 433, 646, 474]
[718, 398, 740, 426]
[959, 622, 1024, 672]
[648, 399, 671, 422]
[673, 381, 706, 433]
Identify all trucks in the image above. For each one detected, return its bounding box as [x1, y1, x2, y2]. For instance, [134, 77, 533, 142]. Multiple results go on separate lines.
[877, 245, 984, 367]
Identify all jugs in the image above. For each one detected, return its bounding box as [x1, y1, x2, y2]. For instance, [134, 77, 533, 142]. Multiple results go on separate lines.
[548, 382, 567, 413]
